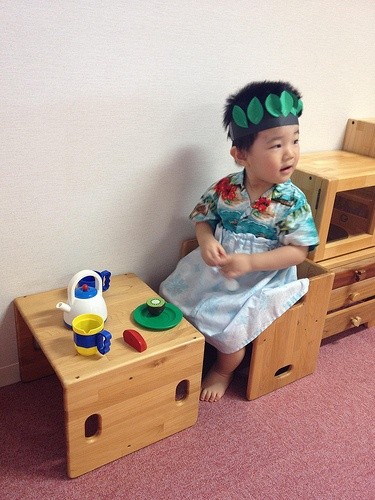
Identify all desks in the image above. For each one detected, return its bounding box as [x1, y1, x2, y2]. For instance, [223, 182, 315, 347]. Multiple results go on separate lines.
[13, 272, 204, 478]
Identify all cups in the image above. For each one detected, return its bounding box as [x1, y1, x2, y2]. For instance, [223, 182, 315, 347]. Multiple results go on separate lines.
[78, 270, 111, 292]
[72, 313, 112, 356]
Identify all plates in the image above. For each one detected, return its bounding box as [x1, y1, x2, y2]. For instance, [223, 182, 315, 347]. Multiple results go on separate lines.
[133, 303, 183, 331]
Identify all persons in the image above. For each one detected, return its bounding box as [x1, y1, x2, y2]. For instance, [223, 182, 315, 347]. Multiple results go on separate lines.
[159, 79, 320, 403]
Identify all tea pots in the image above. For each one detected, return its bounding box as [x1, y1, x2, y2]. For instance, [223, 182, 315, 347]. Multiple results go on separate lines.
[56, 270, 108, 330]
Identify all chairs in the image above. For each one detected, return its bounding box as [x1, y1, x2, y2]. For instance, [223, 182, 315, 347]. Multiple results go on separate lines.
[180, 237, 335, 402]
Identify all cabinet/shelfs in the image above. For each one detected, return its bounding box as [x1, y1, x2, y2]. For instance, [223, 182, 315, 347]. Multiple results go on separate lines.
[289, 118, 375, 339]
